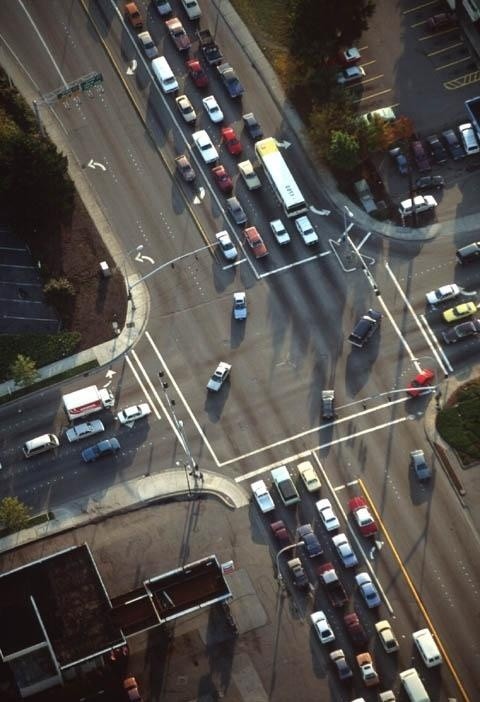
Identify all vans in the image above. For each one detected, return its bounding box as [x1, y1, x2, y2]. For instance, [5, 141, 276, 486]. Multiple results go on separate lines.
[400, 668, 431, 702]
[455, 241, 480, 266]
[413, 627, 442, 669]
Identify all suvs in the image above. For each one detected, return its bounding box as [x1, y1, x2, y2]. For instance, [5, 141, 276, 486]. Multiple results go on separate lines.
[270, 465, 302, 508]
[345, 307, 384, 348]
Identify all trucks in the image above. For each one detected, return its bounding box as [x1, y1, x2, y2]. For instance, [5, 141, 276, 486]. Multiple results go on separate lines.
[61, 386, 117, 423]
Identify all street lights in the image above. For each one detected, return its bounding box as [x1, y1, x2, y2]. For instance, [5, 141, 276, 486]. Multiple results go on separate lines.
[123, 243, 145, 311]
[342, 205, 355, 259]
[275, 541, 306, 580]
[176, 459, 193, 496]
[410, 356, 440, 396]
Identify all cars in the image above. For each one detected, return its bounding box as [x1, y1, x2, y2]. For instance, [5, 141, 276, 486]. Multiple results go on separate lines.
[320, 389, 337, 420]
[426, 280, 480, 345]
[288, 558, 310, 590]
[355, 571, 382, 611]
[120, 673, 145, 702]
[329, 648, 354, 682]
[298, 460, 322, 494]
[205, 361, 232, 393]
[343, 612, 368, 650]
[407, 370, 434, 397]
[349, 495, 378, 538]
[22, 402, 153, 464]
[411, 448, 433, 482]
[297, 524, 323, 558]
[378, 690, 396, 702]
[355, 652, 379, 688]
[310, 610, 335, 645]
[334, 7, 480, 220]
[329, 533, 359, 570]
[316, 498, 340, 532]
[233, 292, 248, 321]
[375, 619, 400, 655]
[250, 479, 276, 517]
[270, 519, 295, 554]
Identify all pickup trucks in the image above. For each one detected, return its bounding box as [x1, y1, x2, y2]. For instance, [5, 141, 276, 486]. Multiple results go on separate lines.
[317, 561, 348, 610]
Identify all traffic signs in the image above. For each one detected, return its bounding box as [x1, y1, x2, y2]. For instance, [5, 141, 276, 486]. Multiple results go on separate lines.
[80, 73, 102, 89]
[58, 86, 79, 99]
